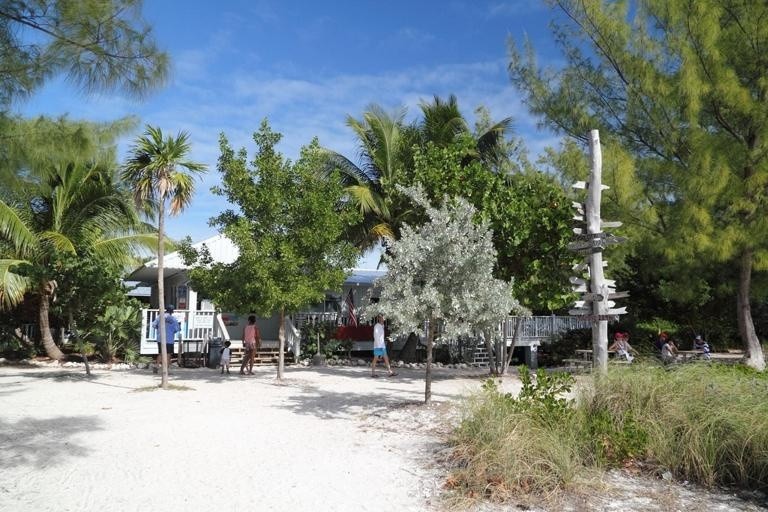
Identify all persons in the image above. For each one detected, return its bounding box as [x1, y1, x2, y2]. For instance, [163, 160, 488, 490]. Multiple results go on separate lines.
[238, 315, 260, 375]
[218, 341, 232, 374]
[609, 331, 710, 365]
[152, 304, 181, 373]
[370, 313, 399, 378]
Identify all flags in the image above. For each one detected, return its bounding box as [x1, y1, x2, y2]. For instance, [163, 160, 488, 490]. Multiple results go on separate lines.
[345, 288, 358, 328]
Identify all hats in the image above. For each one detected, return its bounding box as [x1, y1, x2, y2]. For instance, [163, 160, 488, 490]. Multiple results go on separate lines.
[167, 304, 175, 310]
[615, 332, 631, 338]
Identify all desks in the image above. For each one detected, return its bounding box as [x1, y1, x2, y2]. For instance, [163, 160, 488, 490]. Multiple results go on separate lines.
[576, 350, 615, 361]
[677, 350, 704, 363]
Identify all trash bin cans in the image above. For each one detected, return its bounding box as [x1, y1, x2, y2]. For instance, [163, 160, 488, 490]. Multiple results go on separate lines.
[209, 339, 223, 368]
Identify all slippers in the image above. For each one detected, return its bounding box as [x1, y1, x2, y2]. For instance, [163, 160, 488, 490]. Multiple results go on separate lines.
[389, 373, 398, 377]
[371, 375, 379, 378]
[240, 372, 255, 375]
[153, 367, 158, 374]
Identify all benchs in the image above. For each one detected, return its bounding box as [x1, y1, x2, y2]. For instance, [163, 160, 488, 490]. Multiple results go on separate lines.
[610, 361, 632, 366]
[563, 359, 593, 375]
